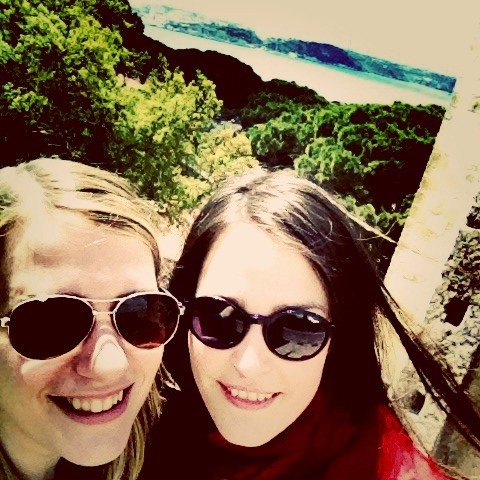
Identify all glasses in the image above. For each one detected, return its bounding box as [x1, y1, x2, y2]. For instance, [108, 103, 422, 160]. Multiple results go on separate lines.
[182, 294, 338, 361]
[0, 284, 186, 361]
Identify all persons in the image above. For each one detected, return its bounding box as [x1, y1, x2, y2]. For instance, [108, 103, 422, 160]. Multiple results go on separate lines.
[0, 157, 187, 480]
[137, 170, 480, 480]
